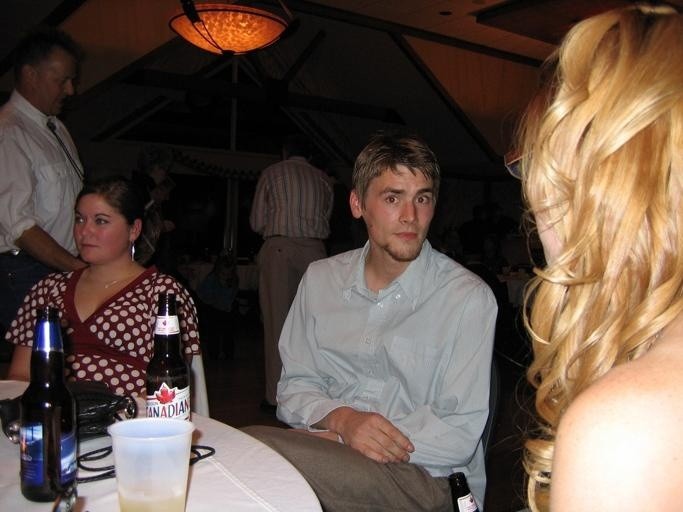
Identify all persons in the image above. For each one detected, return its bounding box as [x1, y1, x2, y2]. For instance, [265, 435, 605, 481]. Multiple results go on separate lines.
[239, 132, 500, 511]
[248, 127, 337, 414]
[5, 166, 203, 401]
[504, 0, 683, 512]
[1, 27, 88, 359]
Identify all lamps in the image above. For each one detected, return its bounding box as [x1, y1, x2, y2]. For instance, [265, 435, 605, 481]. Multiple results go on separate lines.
[166, 1, 295, 65]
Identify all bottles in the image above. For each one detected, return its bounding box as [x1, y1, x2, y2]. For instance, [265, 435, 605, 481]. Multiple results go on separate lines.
[20, 306, 79, 503]
[445, 471, 480, 512]
[144, 290, 193, 420]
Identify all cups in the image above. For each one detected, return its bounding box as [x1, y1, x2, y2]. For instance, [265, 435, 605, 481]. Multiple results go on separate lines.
[106, 418, 196, 512]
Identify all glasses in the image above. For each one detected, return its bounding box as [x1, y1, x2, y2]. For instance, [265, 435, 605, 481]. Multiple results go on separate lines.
[502, 144, 544, 183]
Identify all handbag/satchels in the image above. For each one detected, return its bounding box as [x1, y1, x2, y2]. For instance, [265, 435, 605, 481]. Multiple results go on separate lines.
[0, 380, 130, 444]
